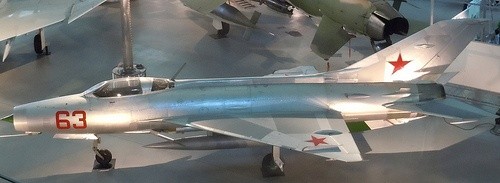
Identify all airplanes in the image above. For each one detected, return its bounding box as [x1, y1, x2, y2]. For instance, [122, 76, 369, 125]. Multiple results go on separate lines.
[180, 0, 411, 61]
[0, 20, 500, 177]
